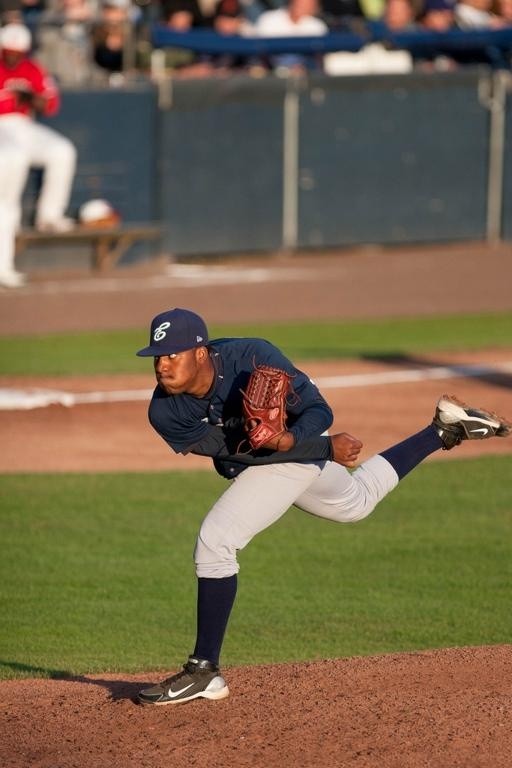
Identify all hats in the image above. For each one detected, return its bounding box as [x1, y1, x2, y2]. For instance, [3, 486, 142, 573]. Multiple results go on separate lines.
[136, 307, 209, 357]
[0, 22, 31, 55]
[79, 198, 121, 231]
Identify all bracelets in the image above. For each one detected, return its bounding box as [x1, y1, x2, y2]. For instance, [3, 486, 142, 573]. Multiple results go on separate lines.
[275, 431, 287, 452]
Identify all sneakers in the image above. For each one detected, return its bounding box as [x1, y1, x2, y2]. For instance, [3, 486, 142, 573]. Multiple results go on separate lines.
[430, 395, 512, 451]
[136, 656, 229, 706]
[0, 214, 75, 290]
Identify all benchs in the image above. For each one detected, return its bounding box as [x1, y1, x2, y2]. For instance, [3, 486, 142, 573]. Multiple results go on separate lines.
[14, 221, 162, 281]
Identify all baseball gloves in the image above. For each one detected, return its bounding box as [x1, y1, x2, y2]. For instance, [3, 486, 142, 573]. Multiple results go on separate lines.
[240, 354, 302, 449]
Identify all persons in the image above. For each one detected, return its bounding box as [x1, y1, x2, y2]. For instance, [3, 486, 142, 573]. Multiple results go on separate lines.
[134, 307, 510, 706]
[0, 1, 512, 94]
[0, 21, 80, 292]
